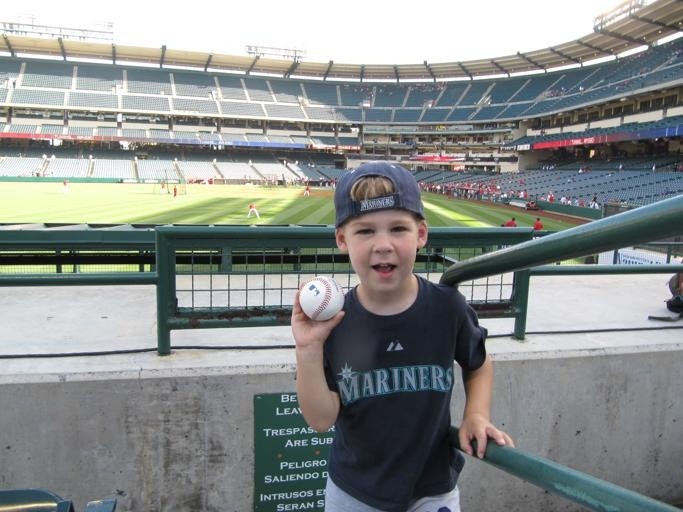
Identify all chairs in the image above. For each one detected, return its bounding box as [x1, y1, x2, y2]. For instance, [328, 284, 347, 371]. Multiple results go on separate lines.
[0, 488, 117, 512]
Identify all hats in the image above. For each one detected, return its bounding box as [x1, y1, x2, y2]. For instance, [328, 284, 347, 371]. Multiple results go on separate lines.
[334, 162, 426, 228]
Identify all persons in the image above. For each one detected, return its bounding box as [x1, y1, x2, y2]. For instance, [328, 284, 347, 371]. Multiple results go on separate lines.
[501, 217, 518, 227]
[290, 164, 513, 511]
[1, 130, 352, 196]
[246, 204, 259, 219]
[352, 42, 683, 214]
[532, 217, 543, 229]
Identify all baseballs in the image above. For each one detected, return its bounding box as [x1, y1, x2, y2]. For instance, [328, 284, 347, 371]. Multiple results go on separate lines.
[298, 276, 345, 322]
